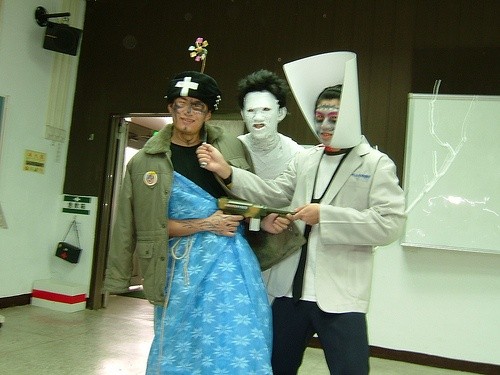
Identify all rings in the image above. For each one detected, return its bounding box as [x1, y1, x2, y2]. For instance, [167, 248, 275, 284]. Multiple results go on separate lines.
[200, 162, 207, 168]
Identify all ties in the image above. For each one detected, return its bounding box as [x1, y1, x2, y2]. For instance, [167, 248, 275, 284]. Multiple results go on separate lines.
[292, 151, 350, 301]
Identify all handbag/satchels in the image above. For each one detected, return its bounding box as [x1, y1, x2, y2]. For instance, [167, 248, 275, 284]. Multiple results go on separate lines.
[56, 220, 81, 264]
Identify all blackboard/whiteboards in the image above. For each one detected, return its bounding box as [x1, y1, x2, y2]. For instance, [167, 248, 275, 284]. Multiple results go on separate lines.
[400, 92, 500, 254]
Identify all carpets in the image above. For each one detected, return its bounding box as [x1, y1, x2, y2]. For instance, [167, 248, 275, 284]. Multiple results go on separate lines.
[117, 290, 145, 299]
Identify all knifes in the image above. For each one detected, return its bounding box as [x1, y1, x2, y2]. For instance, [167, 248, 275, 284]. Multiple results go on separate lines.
[199, 123, 207, 168]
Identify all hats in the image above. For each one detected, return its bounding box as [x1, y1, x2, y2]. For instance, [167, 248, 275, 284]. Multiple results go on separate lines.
[166, 71, 223, 112]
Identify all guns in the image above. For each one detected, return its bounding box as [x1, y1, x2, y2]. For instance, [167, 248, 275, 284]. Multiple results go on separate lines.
[215, 198, 297, 236]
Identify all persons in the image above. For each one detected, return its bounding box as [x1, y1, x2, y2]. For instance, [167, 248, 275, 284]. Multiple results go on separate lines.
[195, 84, 405, 375]
[235, 68, 310, 304]
[101, 69, 272, 375]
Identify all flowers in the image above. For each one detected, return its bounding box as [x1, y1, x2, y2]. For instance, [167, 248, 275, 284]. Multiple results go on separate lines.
[188, 37, 209, 73]
